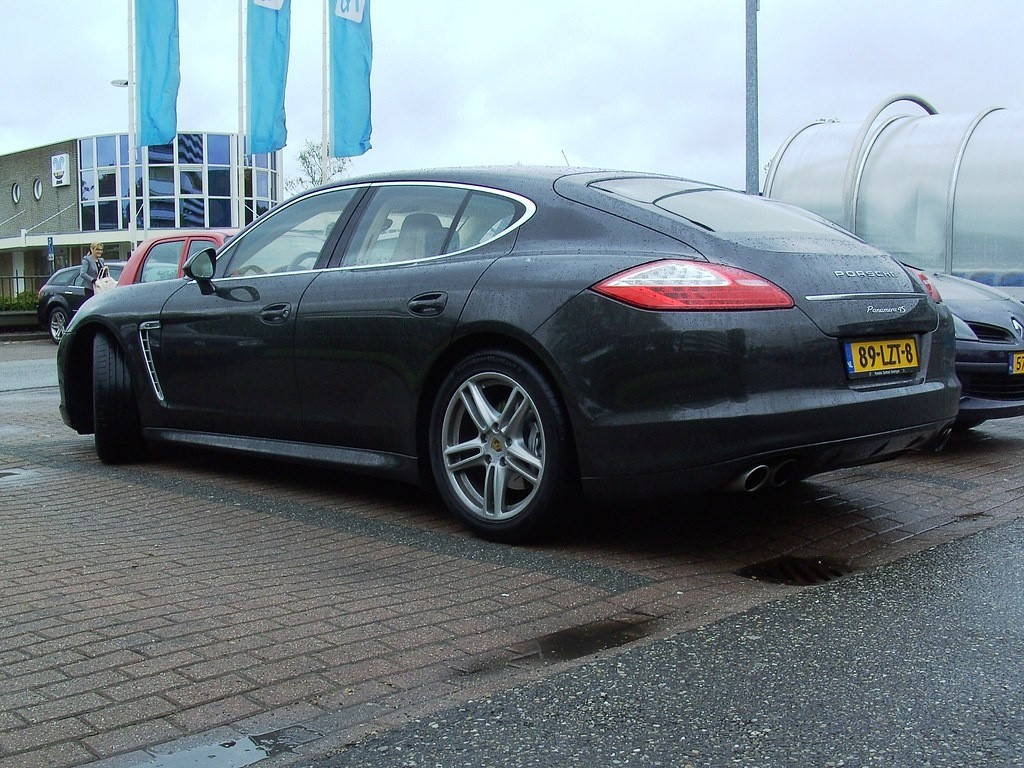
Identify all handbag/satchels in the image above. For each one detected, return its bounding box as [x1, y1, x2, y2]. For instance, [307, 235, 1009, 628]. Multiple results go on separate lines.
[93, 267, 116, 295]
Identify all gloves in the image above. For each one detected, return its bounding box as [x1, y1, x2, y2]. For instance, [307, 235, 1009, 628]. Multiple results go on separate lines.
[91, 279, 97, 283]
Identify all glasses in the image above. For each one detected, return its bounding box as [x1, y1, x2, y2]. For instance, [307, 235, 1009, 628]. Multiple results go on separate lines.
[93, 243, 103, 250]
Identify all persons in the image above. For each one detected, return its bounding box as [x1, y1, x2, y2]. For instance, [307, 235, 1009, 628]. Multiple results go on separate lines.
[80, 242, 105, 298]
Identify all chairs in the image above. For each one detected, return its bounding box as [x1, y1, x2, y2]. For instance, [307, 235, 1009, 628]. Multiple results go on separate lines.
[389, 213, 442, 262]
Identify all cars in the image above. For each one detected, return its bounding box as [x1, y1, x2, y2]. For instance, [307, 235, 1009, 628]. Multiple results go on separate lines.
[37, 261, 178, 343]
[56, 165, 966, 539]
[917, 267, 1023, 449]
[117, 228, 328, 288]
